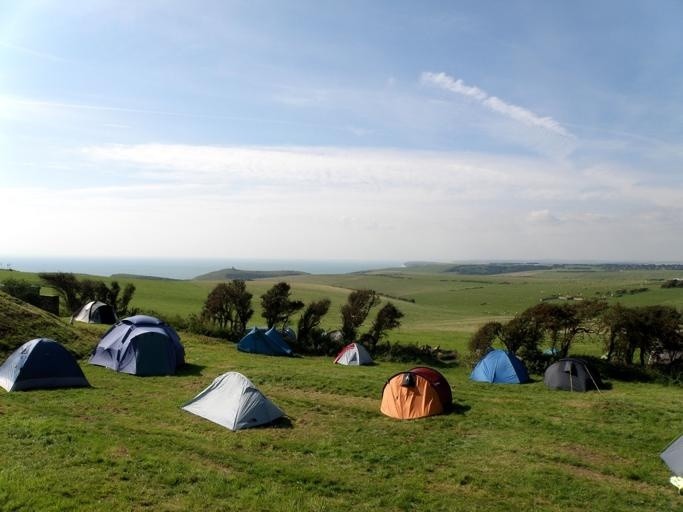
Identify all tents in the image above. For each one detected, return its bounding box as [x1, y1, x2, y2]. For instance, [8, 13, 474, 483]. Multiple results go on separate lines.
[542, 356, 601, 394]
[326, 329, 344, 342]
[467, 349, 529, 386]
[381, 366, 452, 420]
[333, 342, 375, 366]
[88, 314, 186, 376]
[0, 337, 94, 392]
[542, 346, 559, 355]
[657, 428, 683, 493]
[236, 325, 296, 357]
[73, 299, 119, 324]
[179, 370, 295, 433]
